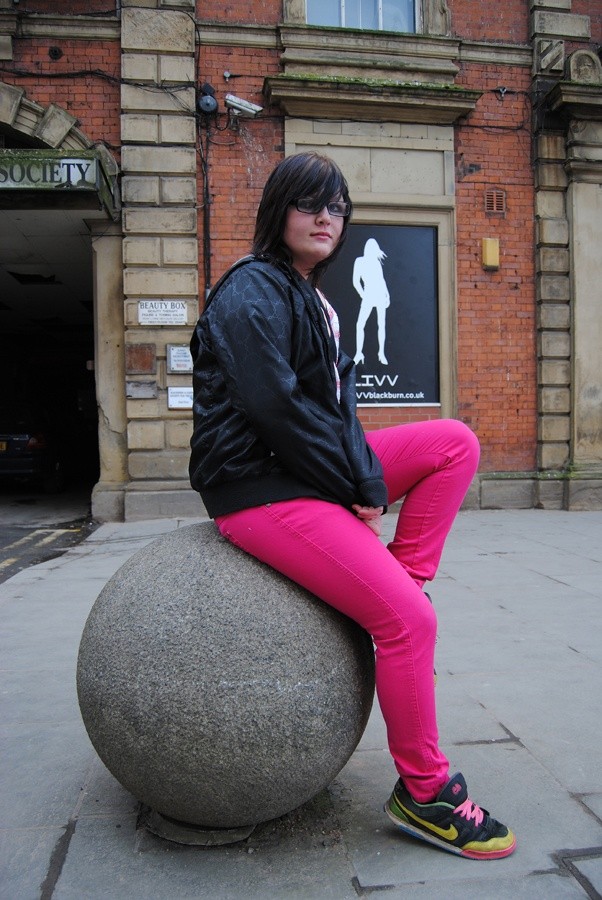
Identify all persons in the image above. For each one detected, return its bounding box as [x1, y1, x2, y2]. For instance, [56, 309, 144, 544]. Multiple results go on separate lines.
[187, 151, 517, 859]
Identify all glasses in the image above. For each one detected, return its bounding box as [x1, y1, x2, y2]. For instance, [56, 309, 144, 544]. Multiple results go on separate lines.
[291, 199, 353, 217]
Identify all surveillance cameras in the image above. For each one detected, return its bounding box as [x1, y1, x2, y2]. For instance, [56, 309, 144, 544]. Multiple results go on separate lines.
[223, 94, 262, 119]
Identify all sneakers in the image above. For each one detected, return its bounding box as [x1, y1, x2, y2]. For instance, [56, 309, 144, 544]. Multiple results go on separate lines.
[382, 764, 517, 860]
